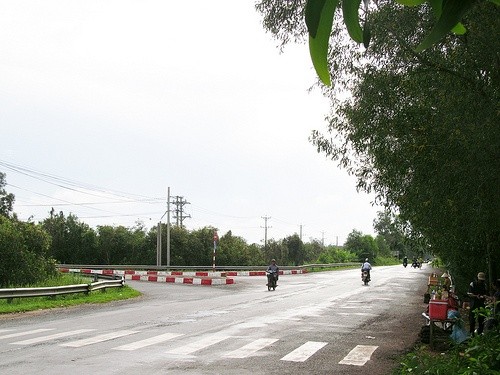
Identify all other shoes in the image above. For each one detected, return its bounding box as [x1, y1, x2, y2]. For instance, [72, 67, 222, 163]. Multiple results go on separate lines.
[471, 332, 475, 337]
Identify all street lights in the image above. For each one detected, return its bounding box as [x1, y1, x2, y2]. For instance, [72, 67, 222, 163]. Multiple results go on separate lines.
[159, 210, 173, 266]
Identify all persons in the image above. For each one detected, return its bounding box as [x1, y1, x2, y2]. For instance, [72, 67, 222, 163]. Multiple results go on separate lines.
[440, 272, 451, 290]
[266, 259, 279, 287]
[411, 258, 417, 267]
[466, 272, 487, 338]
[403, 255, 408, 266]
[362, 258, 372, 281]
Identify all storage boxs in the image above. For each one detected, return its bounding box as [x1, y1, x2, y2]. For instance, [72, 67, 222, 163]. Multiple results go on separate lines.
[430, 298, 448, 302]
[428, 302, 447, 320]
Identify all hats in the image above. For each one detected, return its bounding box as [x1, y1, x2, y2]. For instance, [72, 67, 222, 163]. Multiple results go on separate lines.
[441, 273, 449, 277]
[478, 272, 487, 280]
[272, 259, 276, 262]
[365, 258, 369, 261]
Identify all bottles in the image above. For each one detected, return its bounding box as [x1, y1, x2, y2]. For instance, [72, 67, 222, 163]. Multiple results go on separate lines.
[431, 289, 438, 300]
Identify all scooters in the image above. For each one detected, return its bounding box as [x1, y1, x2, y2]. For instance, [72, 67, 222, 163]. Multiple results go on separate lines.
[266, 270, 278, 291]
[403, 260, 408, 268]
[361, 268, 372, 286]
[412, 260, 421, 268]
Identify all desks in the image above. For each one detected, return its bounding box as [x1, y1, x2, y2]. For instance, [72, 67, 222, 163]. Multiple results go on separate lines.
[427, 317, 459, 350]
[426, 271, 440, 292]
